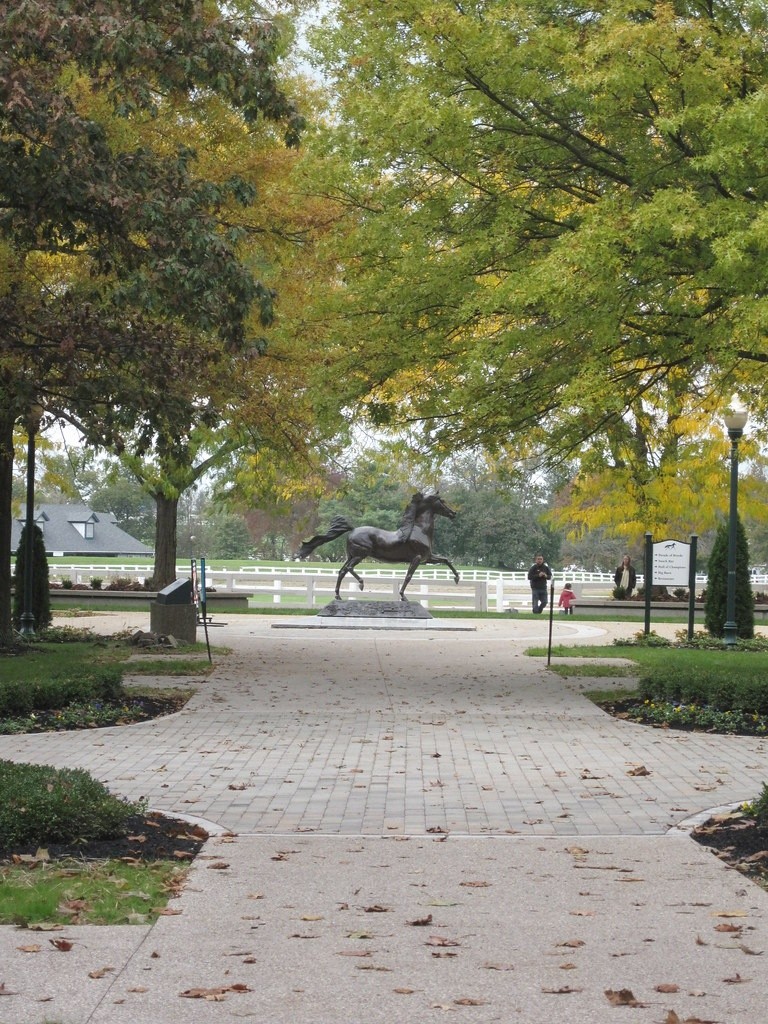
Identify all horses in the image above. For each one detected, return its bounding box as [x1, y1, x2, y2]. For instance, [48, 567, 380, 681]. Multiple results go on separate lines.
[293, 490, 460, 601]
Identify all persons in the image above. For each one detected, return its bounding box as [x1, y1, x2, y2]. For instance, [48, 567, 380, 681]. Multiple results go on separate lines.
[614, 556, 637, 597]
[557, 584, 576, 614]
[528, 554, 553, 614]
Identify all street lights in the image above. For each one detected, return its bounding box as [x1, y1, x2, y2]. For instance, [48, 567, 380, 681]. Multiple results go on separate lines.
[17, 396, 44, 639]
[722, 408, 751, 648]
